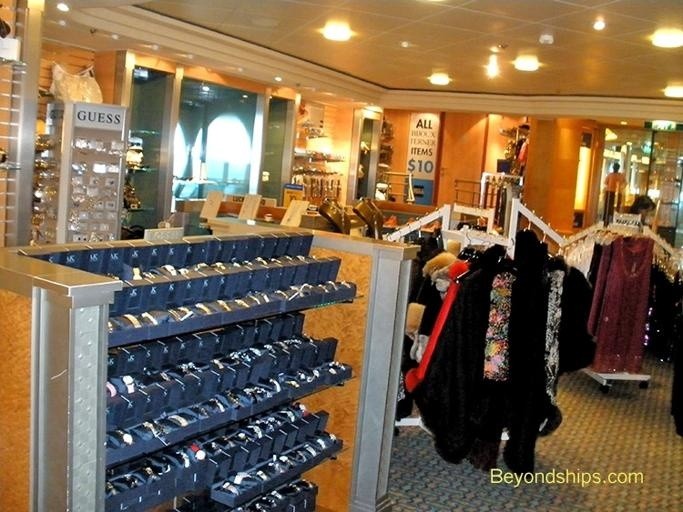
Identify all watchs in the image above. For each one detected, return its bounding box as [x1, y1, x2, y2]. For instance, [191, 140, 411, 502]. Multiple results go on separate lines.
[107, 255, 350, 511]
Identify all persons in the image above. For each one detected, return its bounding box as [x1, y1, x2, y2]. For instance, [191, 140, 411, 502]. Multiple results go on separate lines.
[603, 163, 626, 225]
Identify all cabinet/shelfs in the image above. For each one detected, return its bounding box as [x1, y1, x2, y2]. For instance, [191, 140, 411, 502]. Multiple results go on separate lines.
[293, 154, 345, 176]
[121, 128, 161, 212]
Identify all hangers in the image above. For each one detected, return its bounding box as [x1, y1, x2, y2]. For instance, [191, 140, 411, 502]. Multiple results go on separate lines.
[385, 198, 683, 288]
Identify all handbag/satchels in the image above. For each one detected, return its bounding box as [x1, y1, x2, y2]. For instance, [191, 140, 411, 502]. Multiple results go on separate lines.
[51, 61, 103, 104]
